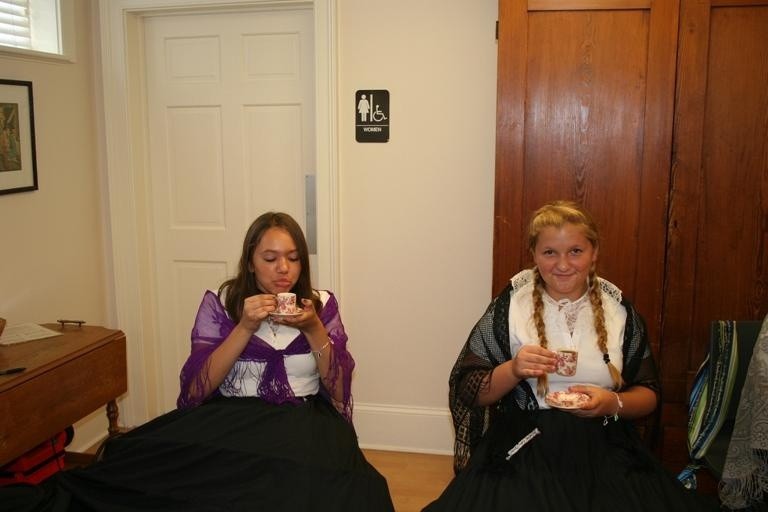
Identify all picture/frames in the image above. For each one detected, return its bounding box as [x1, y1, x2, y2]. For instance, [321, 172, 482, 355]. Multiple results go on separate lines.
[1, 79, 39, 198]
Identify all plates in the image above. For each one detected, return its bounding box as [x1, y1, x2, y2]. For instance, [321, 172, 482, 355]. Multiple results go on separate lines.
[545, 391, 590, 413]
[268, 307, 304, 319]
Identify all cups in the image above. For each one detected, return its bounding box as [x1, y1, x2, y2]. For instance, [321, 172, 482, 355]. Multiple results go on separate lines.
[550, 349, 578, 378]
[272, 291, 297, 314]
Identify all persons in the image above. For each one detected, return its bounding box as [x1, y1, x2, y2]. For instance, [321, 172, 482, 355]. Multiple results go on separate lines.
[176, 211, 353, 423]
[446, 197, 663, 475]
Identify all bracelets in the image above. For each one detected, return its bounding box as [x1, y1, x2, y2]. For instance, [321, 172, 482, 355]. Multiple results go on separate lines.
[602, 390, 624, 427]
[311, 335, 335, 359]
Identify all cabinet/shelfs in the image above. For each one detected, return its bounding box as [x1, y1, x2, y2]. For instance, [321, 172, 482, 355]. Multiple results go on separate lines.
[490, 1, 768, 471]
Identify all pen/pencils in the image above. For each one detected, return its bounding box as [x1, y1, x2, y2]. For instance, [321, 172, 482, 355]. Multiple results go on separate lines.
[0, 367, 26, 374]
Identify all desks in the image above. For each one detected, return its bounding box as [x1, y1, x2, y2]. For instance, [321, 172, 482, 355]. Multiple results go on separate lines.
[0, 320, 130, 468]
